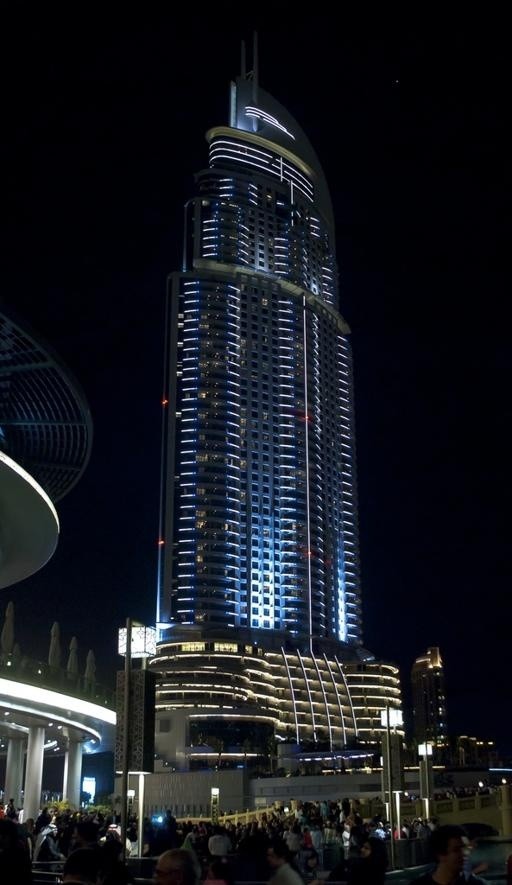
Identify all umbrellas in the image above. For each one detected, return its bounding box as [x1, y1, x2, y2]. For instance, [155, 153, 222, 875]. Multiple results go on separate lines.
[2, 601, 15, 659]
[83, 649, 100, 700]
[47, 620, 62, 670]
[67, 636, 81, 686]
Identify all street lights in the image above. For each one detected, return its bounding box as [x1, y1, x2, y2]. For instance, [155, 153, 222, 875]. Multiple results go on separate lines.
[211, 788, 219, 824]
[381, 705, 406, 866]
[114, 618, 156, 859]
[418, 740, 432, 819]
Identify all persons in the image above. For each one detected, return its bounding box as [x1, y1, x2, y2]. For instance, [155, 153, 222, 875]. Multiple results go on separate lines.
[0, 782, 510, 885]
